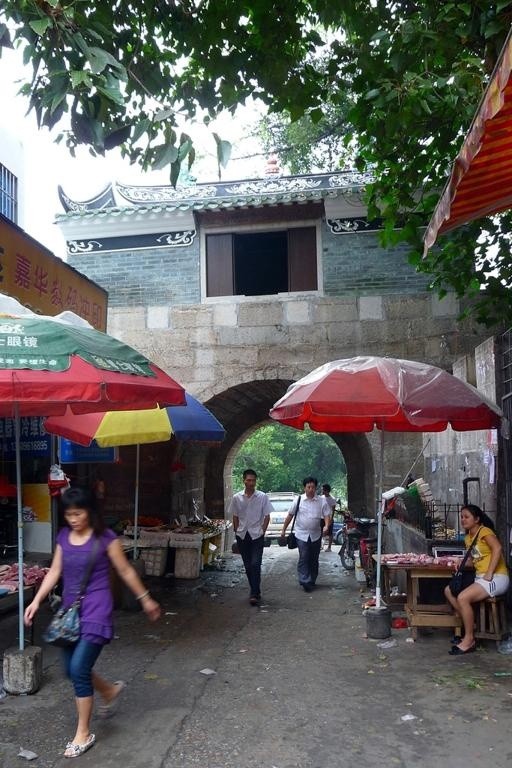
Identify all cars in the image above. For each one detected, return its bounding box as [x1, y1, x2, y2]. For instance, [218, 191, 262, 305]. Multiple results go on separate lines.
[331, 518, 343, 544]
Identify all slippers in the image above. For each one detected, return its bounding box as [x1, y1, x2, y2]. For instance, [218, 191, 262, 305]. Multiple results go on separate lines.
[63, 734, 96, 758]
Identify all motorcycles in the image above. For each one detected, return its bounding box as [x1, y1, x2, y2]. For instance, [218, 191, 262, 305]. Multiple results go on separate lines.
[335, 510, 387, 580]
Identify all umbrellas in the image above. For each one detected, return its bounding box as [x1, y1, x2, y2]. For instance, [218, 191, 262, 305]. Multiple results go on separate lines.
[0, 313, 187, 651]
[44, 392, 226, 561]
[269, 355, 510, 607]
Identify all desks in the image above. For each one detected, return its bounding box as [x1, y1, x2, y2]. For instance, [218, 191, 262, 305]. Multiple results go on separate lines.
[375, 558, 474, 644]
[0, 583, 48, 646]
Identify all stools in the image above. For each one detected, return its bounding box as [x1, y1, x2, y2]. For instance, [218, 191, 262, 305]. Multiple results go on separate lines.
[474, 594, 511, 652]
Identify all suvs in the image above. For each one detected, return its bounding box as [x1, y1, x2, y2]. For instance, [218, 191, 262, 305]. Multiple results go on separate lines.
[263, 491, 301, 548]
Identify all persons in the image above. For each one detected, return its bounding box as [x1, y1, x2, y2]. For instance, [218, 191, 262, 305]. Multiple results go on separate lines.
[281, 477, 332, 592]
[320, 484, 336, 552]
[228, 470, 274, 604]
[24, 487, 161, 758]
[445, 504, 510, 655]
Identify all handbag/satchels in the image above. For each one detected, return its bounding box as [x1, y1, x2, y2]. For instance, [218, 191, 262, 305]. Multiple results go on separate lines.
[288, 533, 298, 549]
[41, 605, 80, 647]
[449, 575, 473, 597]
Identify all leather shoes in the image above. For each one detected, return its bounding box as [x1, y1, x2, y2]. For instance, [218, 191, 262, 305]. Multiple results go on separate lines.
[448, 635, 476, 655]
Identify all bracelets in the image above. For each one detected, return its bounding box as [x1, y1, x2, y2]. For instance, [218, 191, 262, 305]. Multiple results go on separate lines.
[136, 590, 150, 600]
